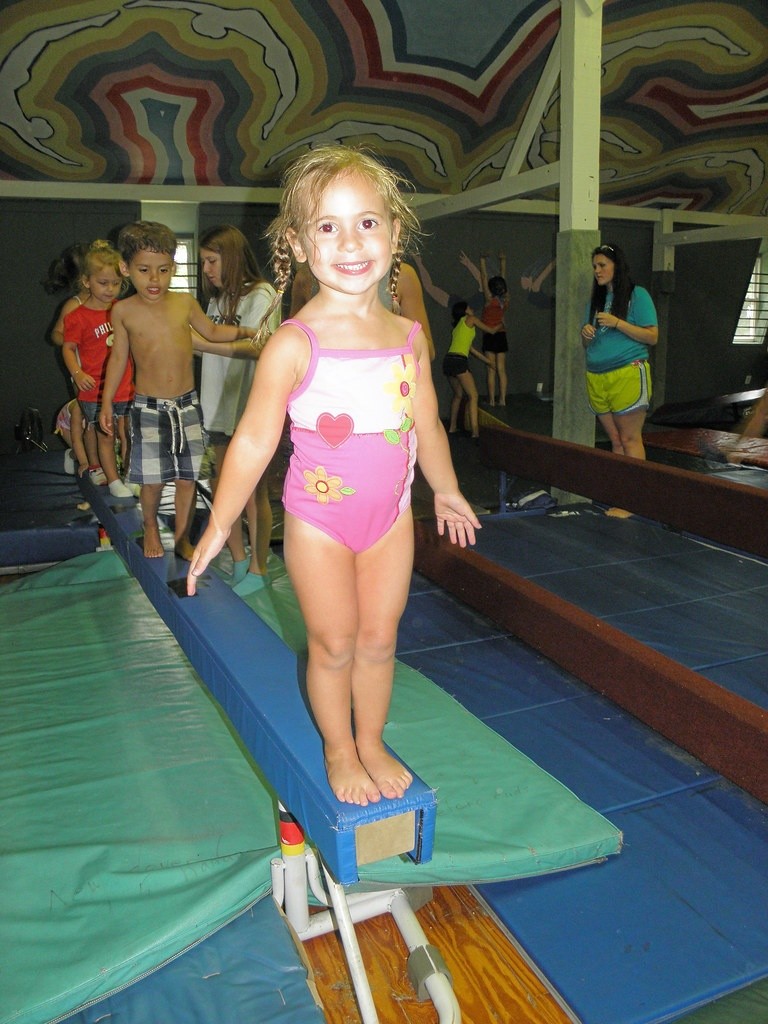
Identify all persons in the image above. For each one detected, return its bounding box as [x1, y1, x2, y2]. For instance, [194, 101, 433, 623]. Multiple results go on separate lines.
[478, 244, 510, 407]
[184, 142, 483, 807]
[580, 241, 660, 520]
[44, 214, 283, 596]
[441, 301, 506, 438]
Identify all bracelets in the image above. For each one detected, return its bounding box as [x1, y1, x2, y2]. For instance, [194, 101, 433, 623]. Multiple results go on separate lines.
[72, 368, 82, 377]
[500, 320, 505, 329]
[614, 318, 622, 329]
[480, 255, 486, 259]
